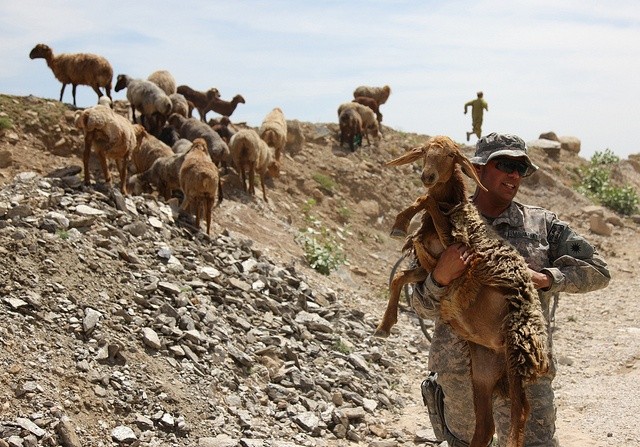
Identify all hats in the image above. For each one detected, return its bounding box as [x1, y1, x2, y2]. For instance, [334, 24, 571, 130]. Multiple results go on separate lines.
[461, 132, 539, 178]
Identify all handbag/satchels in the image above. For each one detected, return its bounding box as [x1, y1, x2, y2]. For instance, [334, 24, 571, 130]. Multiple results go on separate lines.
[421, 376, 448, 441]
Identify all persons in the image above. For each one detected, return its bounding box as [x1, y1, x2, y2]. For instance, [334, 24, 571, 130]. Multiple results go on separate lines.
[464, 91, 489, 142]
[405, 131, 611, 446]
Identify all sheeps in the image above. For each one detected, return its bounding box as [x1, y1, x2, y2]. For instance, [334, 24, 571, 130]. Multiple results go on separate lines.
[206, 93, 246, 121]
[128, 151, 225, 209]
[177, 84, 222, 122]
[339, 106, 362, 152]
[353, 84, 391, 107]
[178, 136, 219, 236]
[209, 115, 241, 146]
[29, 43, 115, 109]
[352, 96, 384, 124]
[338, 101, 384, 147]
[99, 95, 113, 106]
[170, 92, 189, 120]
[188, 100, 196, 119]
[228, 128, 281, 202]
[165, 112, 232, 168]
[172, 137, 194, 153]
[132, 132, 176, 173]
[160, 127, 182, 149]
[258, 106, 288, 162]
[146, 69, 176, 96]
[114, 73, 174, 133]
[371, 134, 557, 447]
[286, 118, 305, 157]
[72, 103, 150, 199]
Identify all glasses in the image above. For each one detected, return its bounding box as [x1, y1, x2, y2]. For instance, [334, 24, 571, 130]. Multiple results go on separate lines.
[491, 157, 531, 177]
